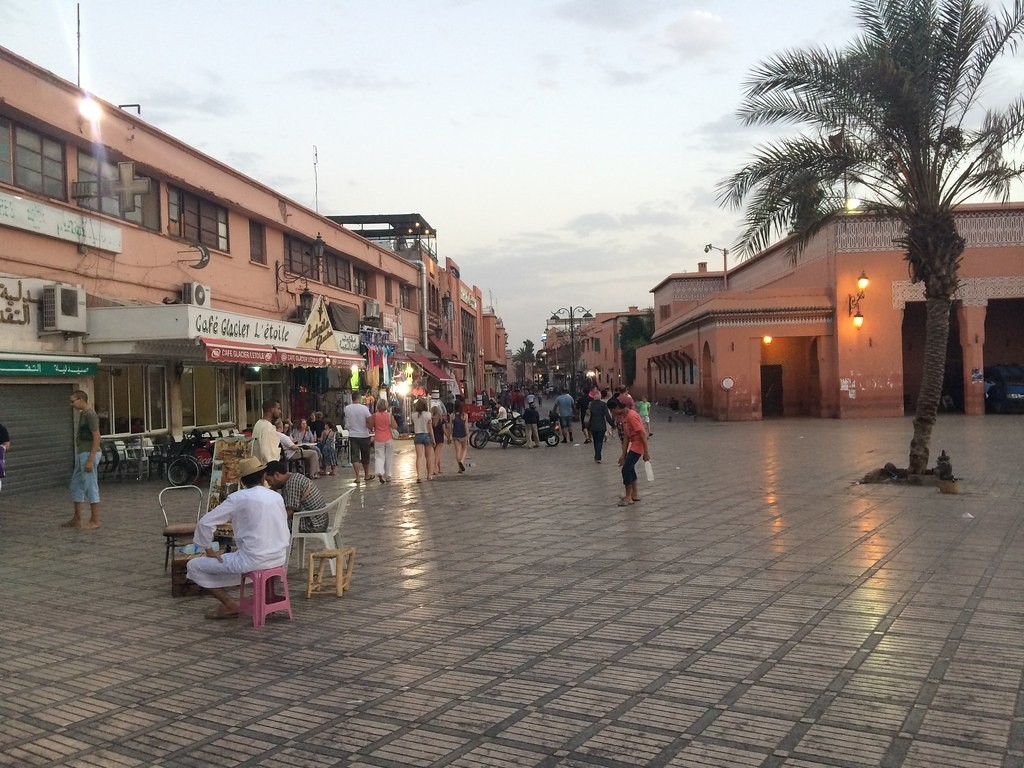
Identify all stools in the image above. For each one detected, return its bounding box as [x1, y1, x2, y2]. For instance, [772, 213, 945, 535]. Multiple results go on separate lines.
[306, 547, 356, 599]
[238, 566, 295, 628]
[171, 548, 226, 597]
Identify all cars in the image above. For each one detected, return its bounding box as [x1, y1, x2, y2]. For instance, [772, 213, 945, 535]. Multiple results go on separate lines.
[985, 363, 1024, 415]
[174, 427, 252, 478]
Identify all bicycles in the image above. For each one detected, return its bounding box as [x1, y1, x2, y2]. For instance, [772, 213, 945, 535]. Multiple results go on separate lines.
[469, 411, 527, 449]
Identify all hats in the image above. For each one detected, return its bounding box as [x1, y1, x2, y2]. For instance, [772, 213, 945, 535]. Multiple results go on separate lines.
[238, 456, 267, 479]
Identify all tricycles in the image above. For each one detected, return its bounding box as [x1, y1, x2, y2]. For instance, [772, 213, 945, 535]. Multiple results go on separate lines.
[141, 431, 206, 487]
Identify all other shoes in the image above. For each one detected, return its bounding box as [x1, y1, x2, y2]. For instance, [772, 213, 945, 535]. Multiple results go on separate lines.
[649, 433, 653, 436]
[632, 496, 640, 501]
[561, 438, 567, 443]
[62, 519, 82, 527]
[205, 605, 239, 619]
[377, 473, 385, 484]
[79, 522, 99, 530]
[596, 458, 601, 463]
[386, 476, 391, 482]
[583, 438, 591, 444]
[618, 498, 634, 506]
[458, 461, 465, 471]
[427, 475, 435, 481]
[433, 468, 442, 475]
[570, 432, 573, 441]
[417, 478, 422, 483]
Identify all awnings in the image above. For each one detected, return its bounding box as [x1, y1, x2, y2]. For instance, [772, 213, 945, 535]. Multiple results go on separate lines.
[408, 353, 454, 382]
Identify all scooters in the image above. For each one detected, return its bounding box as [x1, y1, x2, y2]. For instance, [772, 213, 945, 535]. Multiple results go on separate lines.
[682, 395, 696, 416]
[497, 410, 561, 449]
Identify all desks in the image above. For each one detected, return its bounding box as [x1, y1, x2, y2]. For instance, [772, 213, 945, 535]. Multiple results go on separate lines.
[288, 442, 317, 472]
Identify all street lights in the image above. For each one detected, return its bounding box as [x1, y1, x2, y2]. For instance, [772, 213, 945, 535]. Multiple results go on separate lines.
[704, 244, 728, 291]
[549, 305, 594, 399]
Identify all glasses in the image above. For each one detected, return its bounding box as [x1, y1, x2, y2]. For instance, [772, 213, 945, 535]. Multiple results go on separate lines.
[71, 398, 80, 403]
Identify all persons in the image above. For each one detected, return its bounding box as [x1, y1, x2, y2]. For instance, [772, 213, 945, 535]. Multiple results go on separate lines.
[575, 384, 654, 506]
[184, 455, 291, 618]
[552, 388, 578, 443]
[0, 423, 11, 490]
[499, 382, 554, 413]
[253, 398, 338, 479]
[366, 398, 399, 483]
[265, 460, 328, 533]
[411, 397, 437, 482]
[448, 398, 469, 474]
[61, 390, 104, 529]
[522, 402, 541, 448]
[495, 401, 508, 420]
[344, 391, 377, 483]
[430, 405, 451, 475]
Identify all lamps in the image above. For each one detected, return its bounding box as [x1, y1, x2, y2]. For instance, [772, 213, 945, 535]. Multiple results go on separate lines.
[276, 232, 326, 310]
[430, 292, 449, 322]
[764, 336, 772, 344]
[492, 372, 510, 388]
[849, 270, 869, 328]
[428, 325, 443, 341]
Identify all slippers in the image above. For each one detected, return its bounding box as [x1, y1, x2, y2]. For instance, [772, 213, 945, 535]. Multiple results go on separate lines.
[355, 479, 360, 483]
[364, 474, 375, 480]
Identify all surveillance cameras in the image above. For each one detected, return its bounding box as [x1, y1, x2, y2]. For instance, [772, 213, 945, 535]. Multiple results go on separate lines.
[704, 248, 709, 253]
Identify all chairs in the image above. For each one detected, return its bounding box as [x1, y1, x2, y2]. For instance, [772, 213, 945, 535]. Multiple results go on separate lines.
[278, 425, 350, 478]
[100, 434, 173, 483]
[280, 486, 357, 582]
[158, 486, 203, 571]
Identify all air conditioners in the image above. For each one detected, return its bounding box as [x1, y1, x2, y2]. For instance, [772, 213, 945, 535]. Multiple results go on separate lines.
[366, 299, 380, 318]
[182, 282, 211, 309]
[42, 285, 87, 333]
